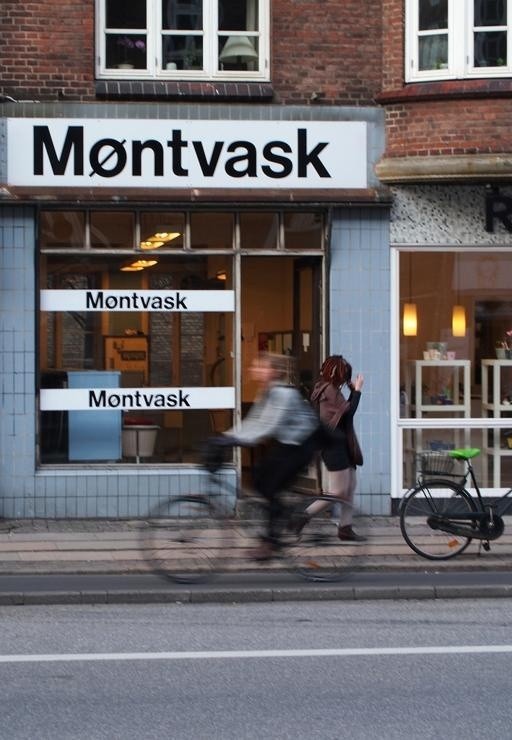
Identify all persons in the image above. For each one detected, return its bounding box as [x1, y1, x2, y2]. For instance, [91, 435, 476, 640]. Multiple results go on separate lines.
[286, 355, 369, 541]
[216, 351, 323, 561]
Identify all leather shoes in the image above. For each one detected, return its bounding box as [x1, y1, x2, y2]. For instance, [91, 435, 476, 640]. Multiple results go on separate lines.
[338, 523, 367, 541]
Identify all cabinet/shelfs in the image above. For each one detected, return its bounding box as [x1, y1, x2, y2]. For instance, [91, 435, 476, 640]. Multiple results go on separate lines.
[480, 359, 512, 488]
[405, 356, 473, 488]
[102, 335, 148, 383]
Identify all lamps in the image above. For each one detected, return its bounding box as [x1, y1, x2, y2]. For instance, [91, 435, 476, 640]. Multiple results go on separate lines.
[402, 251, 419, 339]
[451, 252, 468, 339]
[218, 32, 258, 71]
[119, 216, 179, 276]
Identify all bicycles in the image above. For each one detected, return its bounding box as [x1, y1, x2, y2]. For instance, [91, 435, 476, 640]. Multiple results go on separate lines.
[140, 433, 373, 587]
[396, 429, 512, 563]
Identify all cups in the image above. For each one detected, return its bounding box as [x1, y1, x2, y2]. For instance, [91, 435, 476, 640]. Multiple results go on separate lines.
[422, 341, 456, 360]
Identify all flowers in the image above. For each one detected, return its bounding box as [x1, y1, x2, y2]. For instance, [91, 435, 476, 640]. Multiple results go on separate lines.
[496, 329, 511, 350]
[116, 36, 146, 64]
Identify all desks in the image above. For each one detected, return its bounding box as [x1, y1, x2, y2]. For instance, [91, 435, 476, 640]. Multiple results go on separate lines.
[36, 368, 121, 464]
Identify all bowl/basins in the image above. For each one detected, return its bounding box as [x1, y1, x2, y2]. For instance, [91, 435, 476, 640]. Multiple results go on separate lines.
[494, 348, 511, 359]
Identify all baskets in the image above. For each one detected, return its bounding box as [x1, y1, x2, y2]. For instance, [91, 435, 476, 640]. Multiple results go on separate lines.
[421, 451, 454, 473]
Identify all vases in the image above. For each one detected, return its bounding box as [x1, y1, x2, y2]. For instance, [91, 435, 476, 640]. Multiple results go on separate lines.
[493, 347, 506, 360]
[117, 63, 134, 69]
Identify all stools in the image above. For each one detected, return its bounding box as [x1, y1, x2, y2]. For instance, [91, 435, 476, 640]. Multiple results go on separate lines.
[120, 417, 163, 464]
